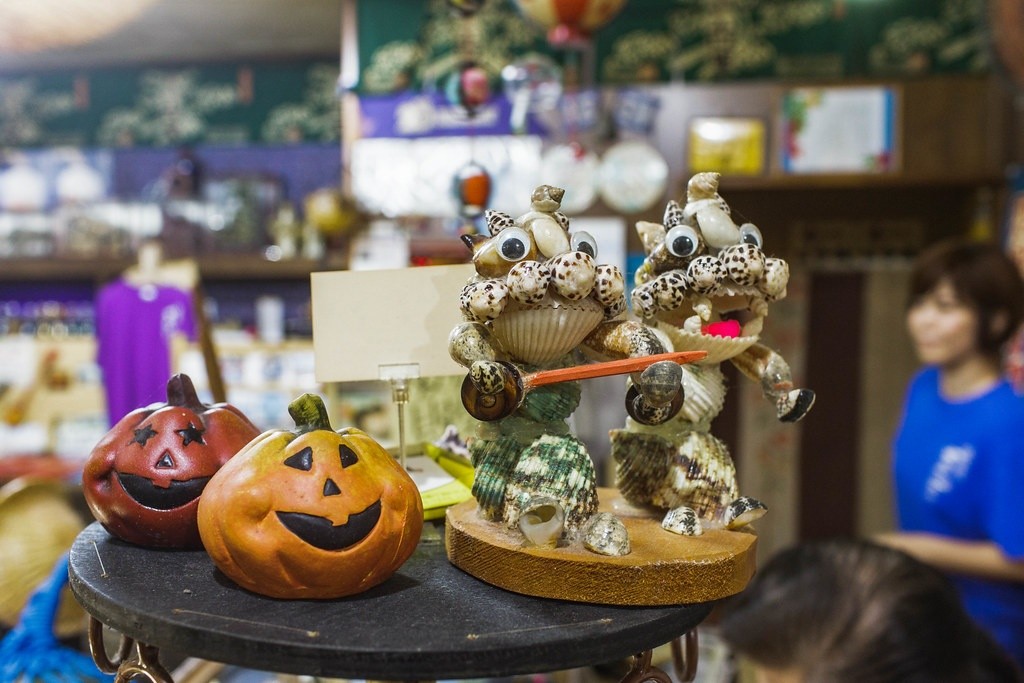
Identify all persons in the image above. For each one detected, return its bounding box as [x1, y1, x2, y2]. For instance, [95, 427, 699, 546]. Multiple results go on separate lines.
[715, 536, 1024, 683]
[863, 238, 1024, 656]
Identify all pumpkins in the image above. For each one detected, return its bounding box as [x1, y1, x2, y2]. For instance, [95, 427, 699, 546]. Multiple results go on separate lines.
[196, 394, 425, 599]
[81, 373, 267, 548]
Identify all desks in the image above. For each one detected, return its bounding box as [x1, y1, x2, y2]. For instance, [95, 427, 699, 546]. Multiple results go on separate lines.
[69, 517, 729, 683]
